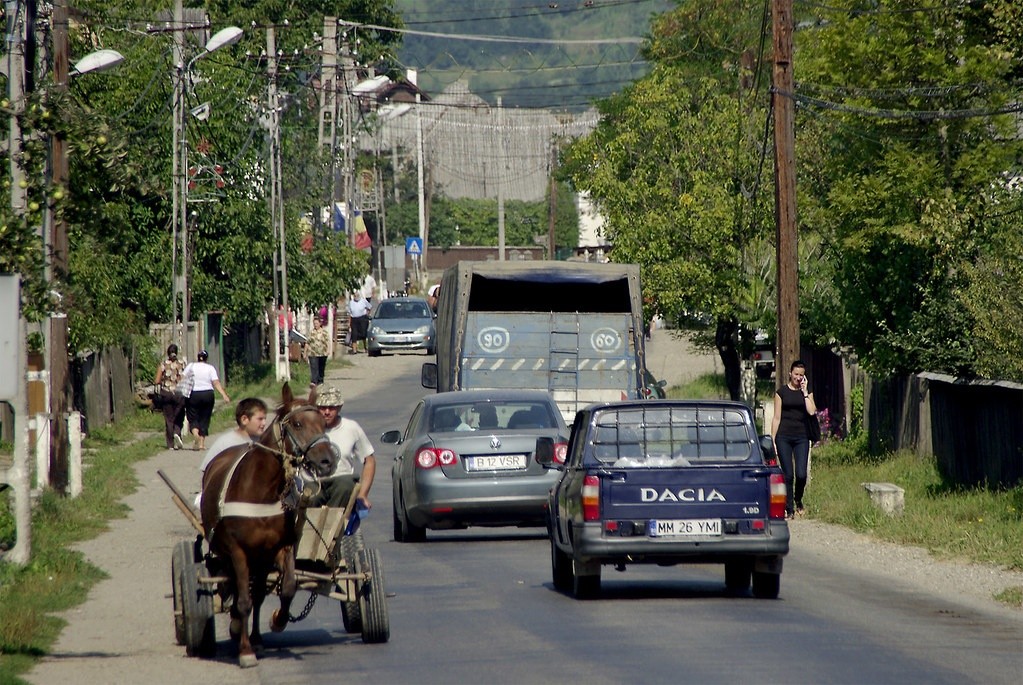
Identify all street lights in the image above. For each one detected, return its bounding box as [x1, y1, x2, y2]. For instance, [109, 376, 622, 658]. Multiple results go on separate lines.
[371, 103, 413, 299]
[182, 24, 244, 370]
[329, 74, 392, 227]
[49, 48, 124, 495]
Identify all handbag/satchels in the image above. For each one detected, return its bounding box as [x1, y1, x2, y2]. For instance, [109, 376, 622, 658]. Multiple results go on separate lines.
[150, 384, 164, 410]
[175, 370, 194, 398]
[345, 328, 352, 345]
[806, 414, 820, 442]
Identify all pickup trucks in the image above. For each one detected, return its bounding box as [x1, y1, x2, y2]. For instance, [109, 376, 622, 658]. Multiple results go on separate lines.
[535, 398, 790, 599]
[752, 331, 775, 378]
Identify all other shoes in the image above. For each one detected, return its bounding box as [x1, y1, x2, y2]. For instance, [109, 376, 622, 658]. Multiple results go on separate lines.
[309, 383, 315, 390]
[192, 435, 200, 451]
[199, 445, 210, 450]
[174, 434, 183, 448]
[785, 514, 794, 521]
[797, 508, 803, 515]
[169, 448, 174, 451]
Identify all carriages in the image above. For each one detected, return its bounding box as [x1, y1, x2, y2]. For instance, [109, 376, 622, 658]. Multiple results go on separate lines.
[172, 382, 389, 669]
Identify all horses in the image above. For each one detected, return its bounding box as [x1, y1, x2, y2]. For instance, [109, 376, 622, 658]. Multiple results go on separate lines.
[198, 379, 341, 668]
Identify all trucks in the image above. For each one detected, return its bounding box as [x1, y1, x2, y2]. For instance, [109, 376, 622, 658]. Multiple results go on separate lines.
[420, 260, 646, 426]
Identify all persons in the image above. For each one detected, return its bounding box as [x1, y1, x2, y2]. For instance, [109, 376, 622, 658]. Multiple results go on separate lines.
[348, 292, 372, 354]
[771, 360, 817, 521]
[200, 398, 267, 472]
[155, 344, 186, 451]
[360, 274, 377, 316]
[298, 382, 376, 512]
[303, 318, 331, 389]
[180, 350, 230, 451]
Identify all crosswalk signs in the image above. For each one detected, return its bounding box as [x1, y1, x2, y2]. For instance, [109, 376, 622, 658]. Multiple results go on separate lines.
[405, 237, 422, 253]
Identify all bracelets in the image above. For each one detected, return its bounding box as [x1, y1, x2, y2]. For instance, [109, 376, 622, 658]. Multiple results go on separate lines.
[804, 396, 808, 398]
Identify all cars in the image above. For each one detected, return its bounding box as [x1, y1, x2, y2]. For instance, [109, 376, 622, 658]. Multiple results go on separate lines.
[364, 297, 439, 356]
[380, 390, 572, 542]
[643, 369, 666, 399]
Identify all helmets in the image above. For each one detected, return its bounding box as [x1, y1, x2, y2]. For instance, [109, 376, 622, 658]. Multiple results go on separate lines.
[314, 383, 344, 406]
